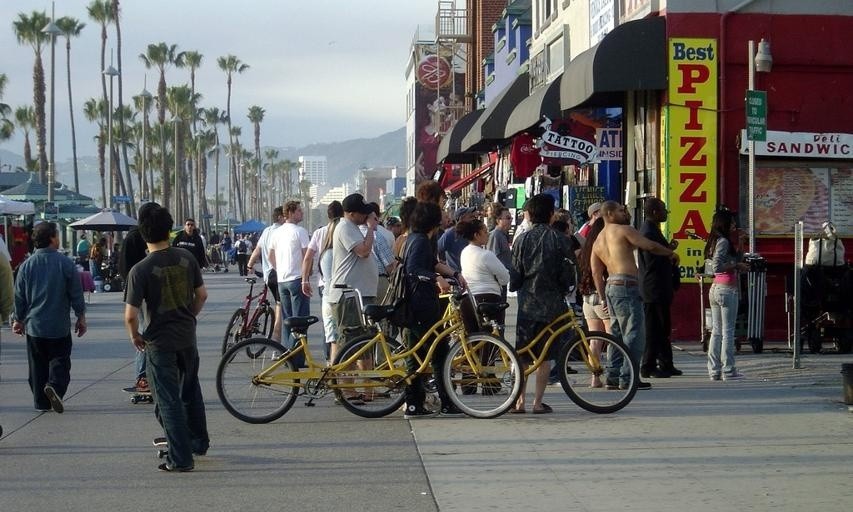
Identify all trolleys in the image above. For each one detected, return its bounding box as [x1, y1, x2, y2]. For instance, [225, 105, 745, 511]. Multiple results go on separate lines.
[204, 243, 224, 273]
[696, 254, 766, 354]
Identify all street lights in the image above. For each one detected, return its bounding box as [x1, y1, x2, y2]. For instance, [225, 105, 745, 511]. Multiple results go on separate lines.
[101, 48, 120, 263]
[194, 128, 288, 237]
[136, 73, 149, 208]
[41, 1, 64, 225]
[170, 102, 182, 228]
[743, 35, 772, 258]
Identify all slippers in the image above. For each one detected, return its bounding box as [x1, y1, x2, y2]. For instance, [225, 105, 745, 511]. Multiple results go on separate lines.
[533, 403, 552, 413]
[334, 394, 372, 405]
[508, 405, 525, 412]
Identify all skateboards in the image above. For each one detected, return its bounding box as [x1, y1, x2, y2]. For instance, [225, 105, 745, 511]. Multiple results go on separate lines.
[153, 437, 169, 457]
[121, 387, 152, 403]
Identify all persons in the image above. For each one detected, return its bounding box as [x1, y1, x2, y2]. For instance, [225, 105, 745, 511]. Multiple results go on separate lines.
[244, 205, 286, 360]
[296, 180, 683, 421]
[118, 225, 153, 389]
[415, 92, 465, 180]
[71, 228, 271, 293]
[0, 252, 17, 438]
[11, 220, 87, 413]
[170, 216, 208, 271]
[267, 200, 313, 368]
[414, 94, 451, 180]
[702, 210, 752, 382]
[123, 203, 208, 473]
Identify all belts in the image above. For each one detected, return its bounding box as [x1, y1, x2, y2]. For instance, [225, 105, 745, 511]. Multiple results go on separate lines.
[607, 280, 636, 285]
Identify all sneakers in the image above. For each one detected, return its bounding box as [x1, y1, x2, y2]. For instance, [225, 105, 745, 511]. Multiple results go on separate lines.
[567, 366, 682, 390]
[158, 463, 193, 471]
[404, 403, 465, 420]
[124, 387, 136, 392]
[38, 384, 63, 412]
[711, 372, 743, 379]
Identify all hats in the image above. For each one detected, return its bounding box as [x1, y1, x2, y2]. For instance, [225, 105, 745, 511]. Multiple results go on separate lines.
[455, 207, 475, 220]
[588, 202, 602, 218]
[343, 194, 371, 212]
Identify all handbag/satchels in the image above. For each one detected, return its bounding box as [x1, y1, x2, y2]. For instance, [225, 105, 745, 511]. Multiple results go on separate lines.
[806, 239, 845, 266]
[381, 256, 417, 351]
